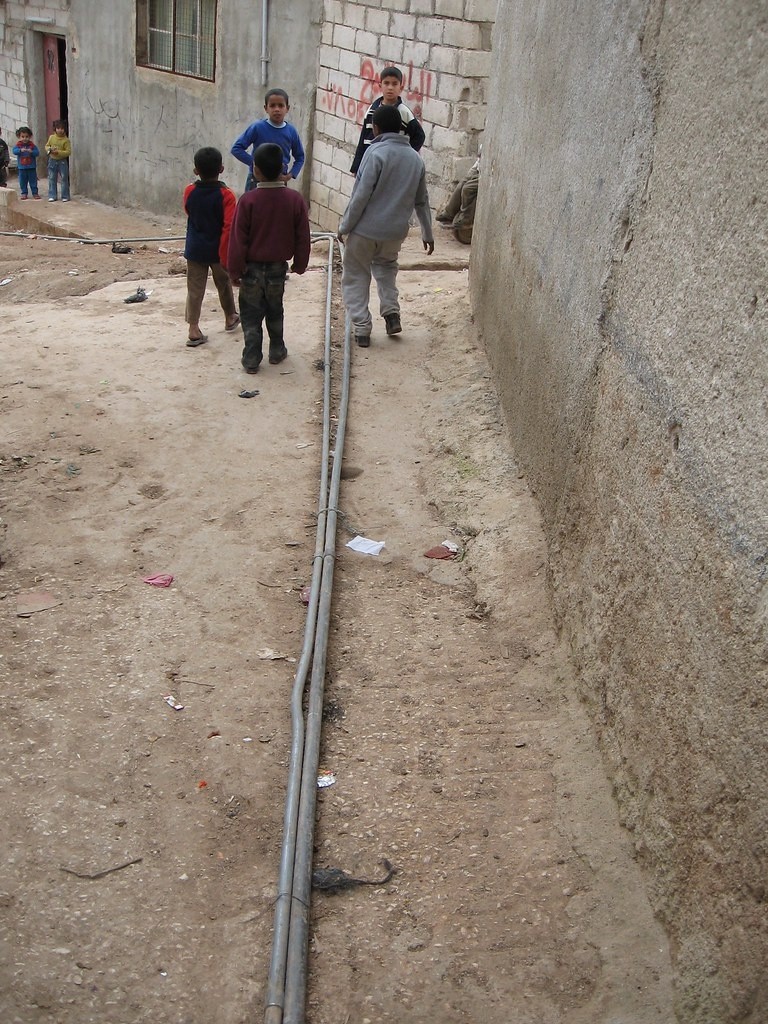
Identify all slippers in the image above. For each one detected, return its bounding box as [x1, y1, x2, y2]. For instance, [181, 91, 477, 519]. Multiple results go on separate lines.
[185, 331, 208, 346]
[225, 312, 241, 331]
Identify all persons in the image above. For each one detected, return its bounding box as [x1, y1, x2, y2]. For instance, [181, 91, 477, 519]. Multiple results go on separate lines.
[0, 127, 10, 187]
[12, 127, 41, 200]
[44, 119, 72, 202]
[350, 67, 426, 175]
[227, 144, 311, 374]
[231, 88, 305, 193]
[338, 106, 434, 347]
[183, 146, 241, 346]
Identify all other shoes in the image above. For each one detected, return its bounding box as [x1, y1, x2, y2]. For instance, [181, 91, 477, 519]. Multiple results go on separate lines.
[355, 335, 370, 349]
[269, 346, 287, 364]
[62, 198, 68, 202]
[383, 312, 403, 333]
[49, 197, 54, 202]
[34, 195, 41, 199]
[241, 357, 259, 374]
[20, 196, 28, 200]
